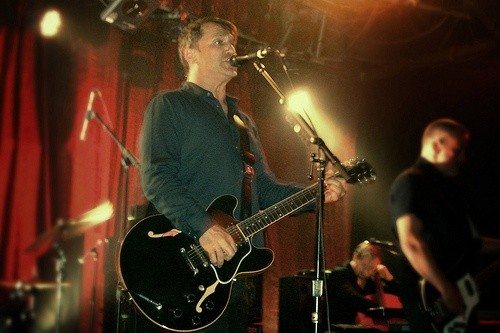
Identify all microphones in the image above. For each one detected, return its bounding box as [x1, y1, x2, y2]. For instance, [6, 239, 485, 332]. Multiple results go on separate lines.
[370, 237, 394, 246]
[80, 89, 96, 141]
[230, 48, 274, 67]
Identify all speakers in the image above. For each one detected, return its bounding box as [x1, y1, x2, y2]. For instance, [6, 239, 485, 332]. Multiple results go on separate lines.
[279, 269, 328, 333]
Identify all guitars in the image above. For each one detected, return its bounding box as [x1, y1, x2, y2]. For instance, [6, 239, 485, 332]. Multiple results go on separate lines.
[115, 156, 376, 333]
[419, 258, 500, 333]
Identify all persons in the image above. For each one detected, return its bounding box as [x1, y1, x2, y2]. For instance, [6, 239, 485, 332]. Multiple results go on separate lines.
[139, 14, 350, 333]
[322, 240, 401, 333]
[389, 118, 482, 333]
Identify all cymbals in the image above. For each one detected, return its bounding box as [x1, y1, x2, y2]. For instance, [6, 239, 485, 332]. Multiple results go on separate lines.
[371, 238, 404, 256]
